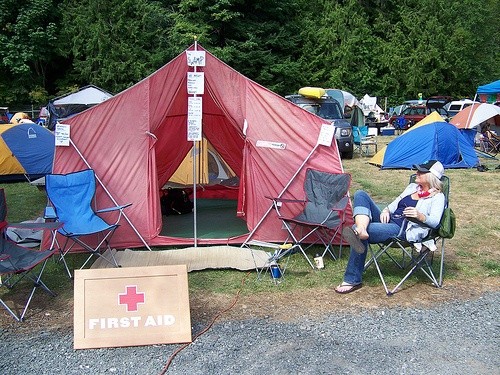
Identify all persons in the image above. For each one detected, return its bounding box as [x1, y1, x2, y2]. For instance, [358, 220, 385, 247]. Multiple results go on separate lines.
[478, 120, 491, 154]
[333, 159, 445, 293]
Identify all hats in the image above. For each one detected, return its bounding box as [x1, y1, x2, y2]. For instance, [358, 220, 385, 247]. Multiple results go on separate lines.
[412, 159, 444, 180]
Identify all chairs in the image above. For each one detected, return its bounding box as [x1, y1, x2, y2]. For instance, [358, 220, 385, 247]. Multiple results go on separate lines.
[365, 174, 450, 295]
[264, 167, 352, 270]
[46, 168, 133, 280]
[0, 187, 65, 322]
[353, 126, 378, 157]
[476, 131, 500, 154]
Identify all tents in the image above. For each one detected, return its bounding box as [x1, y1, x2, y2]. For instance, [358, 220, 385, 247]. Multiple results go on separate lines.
[365, 110, 447, 165]
[465, 80, 500, 129]
[0, 124, 55, 179]
[449, 103, 500, 146]
[39, 35, 356, 254]
[380, 122, 480, 170]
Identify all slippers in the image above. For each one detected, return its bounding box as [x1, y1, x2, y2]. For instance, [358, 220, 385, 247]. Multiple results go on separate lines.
[333, 281, 363, 294]
[343, 226, 366, 254]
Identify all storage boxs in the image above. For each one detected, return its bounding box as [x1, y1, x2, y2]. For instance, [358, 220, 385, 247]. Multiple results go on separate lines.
[382, 128, 396, 135]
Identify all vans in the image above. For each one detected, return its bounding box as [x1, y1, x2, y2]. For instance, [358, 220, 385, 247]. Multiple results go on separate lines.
[449, 101, 479, 117]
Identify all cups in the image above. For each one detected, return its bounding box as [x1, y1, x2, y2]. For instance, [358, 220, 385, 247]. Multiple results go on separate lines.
[313, 253, 325, 269]
[270, 264, 281, 279]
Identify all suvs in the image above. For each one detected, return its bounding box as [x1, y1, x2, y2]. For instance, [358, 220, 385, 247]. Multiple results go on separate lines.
[286, 95, 352, 159]
[391, 96, 448, 129]
